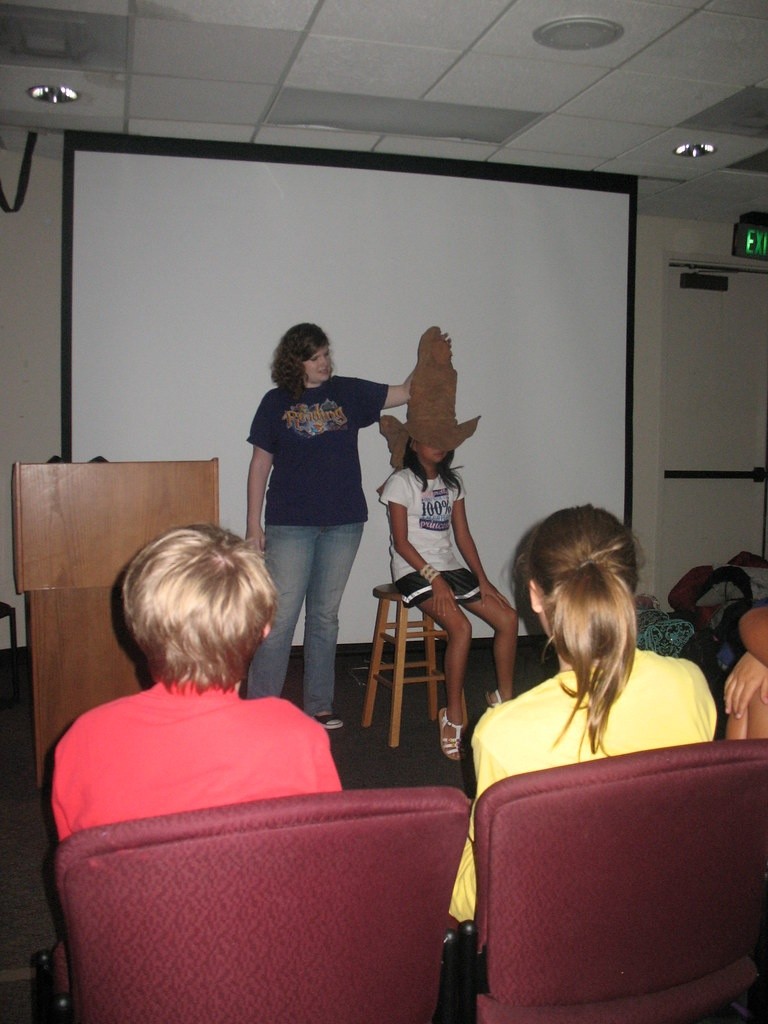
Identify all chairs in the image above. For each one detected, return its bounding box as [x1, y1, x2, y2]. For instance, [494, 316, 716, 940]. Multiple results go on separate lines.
[36, 788, 469, 1024]
[456, 737, 768, 1024]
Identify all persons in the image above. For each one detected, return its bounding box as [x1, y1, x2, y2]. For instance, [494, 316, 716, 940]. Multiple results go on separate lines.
[244, 322, 453, 728]
[379, 436, 519, 762]
[49, 524, 342, 843]
[723, 606, 768, 739]
[445, 502, 717, 922]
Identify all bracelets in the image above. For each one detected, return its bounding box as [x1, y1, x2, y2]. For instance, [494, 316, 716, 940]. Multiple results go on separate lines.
[420, 564, 443, 586]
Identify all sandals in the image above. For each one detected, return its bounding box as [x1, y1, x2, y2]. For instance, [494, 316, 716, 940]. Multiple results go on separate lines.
[485, 689, 502, 708]
[439, 707, 468, 761]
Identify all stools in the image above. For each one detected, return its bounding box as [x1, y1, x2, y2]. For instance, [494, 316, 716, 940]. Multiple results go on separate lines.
[362, 583, 468, 746]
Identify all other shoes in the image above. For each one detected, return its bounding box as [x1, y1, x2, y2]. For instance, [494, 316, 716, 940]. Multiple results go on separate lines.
[315, 711, 344, 729]
